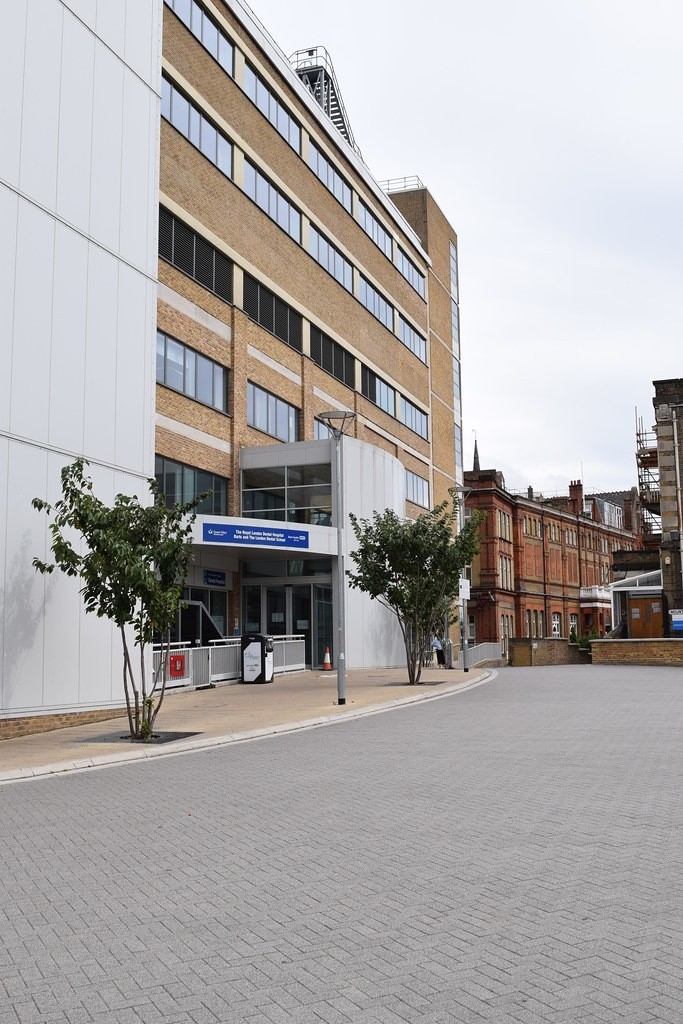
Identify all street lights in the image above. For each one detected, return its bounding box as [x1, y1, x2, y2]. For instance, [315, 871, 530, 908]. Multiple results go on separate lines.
[317, 408, 357, 704]
[448, 485, 473, 672]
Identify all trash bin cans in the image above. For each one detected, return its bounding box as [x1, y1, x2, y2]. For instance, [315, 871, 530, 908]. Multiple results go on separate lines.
[240, 633, 274, 684]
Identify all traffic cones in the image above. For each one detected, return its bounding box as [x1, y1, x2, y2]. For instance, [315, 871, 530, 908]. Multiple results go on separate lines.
[319, 647, 334, 671]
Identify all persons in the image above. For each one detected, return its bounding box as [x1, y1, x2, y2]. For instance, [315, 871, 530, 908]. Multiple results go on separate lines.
[431, 635, 447, 670]
